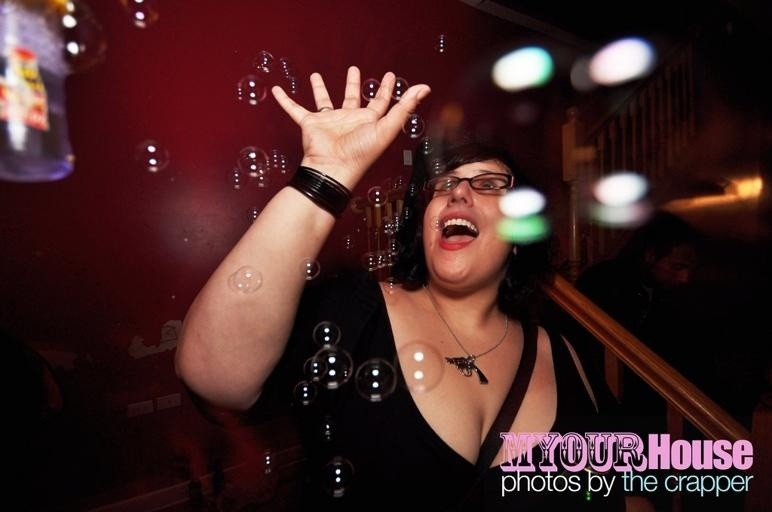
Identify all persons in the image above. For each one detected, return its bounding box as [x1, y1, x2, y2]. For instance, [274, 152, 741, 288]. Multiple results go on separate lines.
[173, 66, 652, 510]
[548, 209, 744, 512]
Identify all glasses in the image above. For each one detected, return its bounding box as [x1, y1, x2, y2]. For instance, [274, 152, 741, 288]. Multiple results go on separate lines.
[422, 172, 514, 203]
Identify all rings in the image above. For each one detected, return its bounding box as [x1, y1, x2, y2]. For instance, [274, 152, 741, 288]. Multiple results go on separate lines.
[317, 105, 333, 112]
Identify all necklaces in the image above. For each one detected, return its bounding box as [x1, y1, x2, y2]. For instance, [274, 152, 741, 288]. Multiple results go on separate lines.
[421, 282, 508, 385]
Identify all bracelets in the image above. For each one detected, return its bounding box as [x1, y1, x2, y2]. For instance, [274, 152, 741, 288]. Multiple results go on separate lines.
[288, 166, 350, 222]
